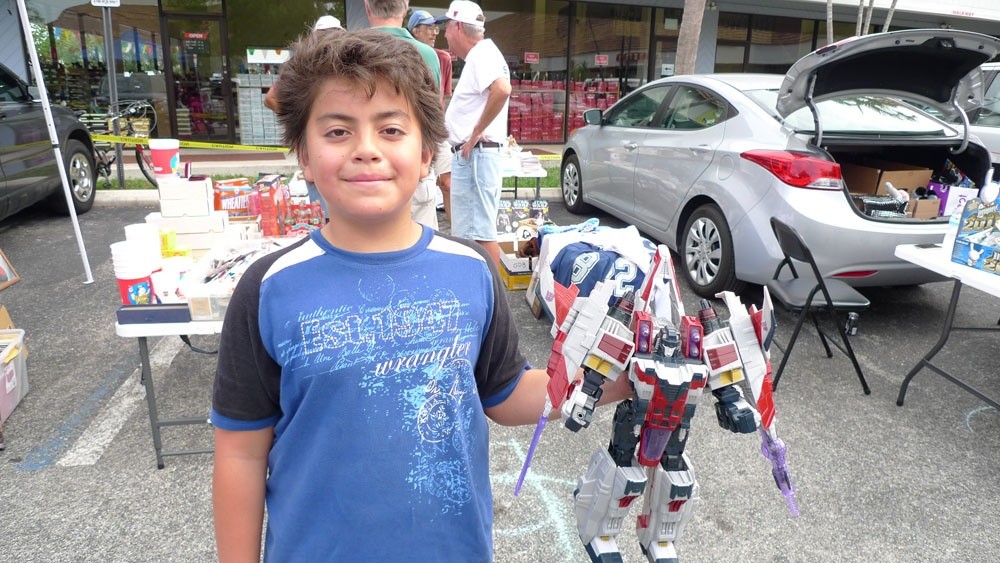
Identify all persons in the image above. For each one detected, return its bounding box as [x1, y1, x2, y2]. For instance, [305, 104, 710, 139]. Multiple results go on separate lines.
[440, 0, 513, 278]
[208, 32, 660, 563]
[354, 0, 441, 236]
[408, 10, 455, 228]
[264, 15, 348, 229]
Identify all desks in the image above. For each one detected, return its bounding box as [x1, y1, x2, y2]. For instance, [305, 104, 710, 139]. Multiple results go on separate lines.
[116, 323, 220, 470]
[500, 168, 548, 201]
[893, 244, 1000, 415]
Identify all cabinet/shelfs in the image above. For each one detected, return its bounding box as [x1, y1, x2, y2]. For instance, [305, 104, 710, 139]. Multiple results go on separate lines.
[41, 59, 110, 140]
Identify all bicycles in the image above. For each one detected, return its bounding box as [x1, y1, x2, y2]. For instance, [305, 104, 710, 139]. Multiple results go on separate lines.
[75, 100, 157, 186]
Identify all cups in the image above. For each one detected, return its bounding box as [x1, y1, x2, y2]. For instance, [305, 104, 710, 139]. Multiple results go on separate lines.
[147, 139, 181, 178]
[111, 222, 194, 309]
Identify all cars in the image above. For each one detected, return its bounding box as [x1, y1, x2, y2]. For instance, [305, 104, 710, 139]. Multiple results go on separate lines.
[0, 63, 95, 224]
[560, 27, 999, 314]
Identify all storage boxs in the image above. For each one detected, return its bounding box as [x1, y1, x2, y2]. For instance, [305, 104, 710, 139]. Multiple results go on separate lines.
[176, 108, 192, 140]
[506, 79, 620, 142]
[840, 161, 980, 219]
[236, 73, 291, 147]
[0, 329, 29, 429]
[497, 242, 538, 291]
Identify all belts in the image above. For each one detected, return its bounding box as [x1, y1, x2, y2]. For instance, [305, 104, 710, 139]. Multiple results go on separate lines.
[451, 141, 502, 154]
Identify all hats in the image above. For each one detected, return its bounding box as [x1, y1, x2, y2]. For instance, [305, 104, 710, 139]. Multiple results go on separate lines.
[313, 15, 347, 31]
[408, 11, 436, 31]
[434, 0, 486, 26]
